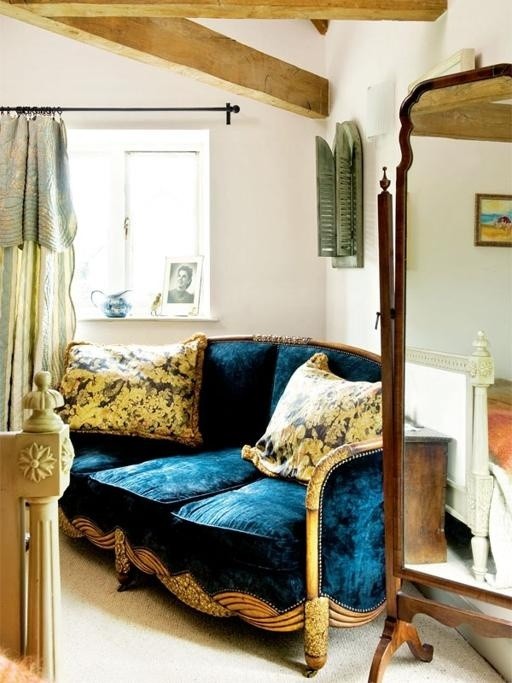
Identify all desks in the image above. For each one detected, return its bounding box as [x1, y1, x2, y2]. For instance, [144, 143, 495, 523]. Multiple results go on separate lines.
[402, 417, 451, 563]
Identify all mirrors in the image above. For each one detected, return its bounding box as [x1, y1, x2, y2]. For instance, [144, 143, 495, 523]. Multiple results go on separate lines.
[368, 63, 512, 683]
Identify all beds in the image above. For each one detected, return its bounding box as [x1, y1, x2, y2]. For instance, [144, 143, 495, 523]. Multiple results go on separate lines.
[403, 330, 512, 585]
[0, 368, 75, 683]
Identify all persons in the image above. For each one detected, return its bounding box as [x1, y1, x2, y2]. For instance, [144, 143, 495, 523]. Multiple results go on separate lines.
[169, 264, 194, 302]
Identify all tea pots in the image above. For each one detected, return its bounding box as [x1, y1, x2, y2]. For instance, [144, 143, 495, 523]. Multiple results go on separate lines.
[90, 287, 135, 318]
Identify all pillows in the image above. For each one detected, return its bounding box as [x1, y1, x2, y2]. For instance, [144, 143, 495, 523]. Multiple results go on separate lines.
[242, 353, 382, 487]
[53, 332, 207, 447]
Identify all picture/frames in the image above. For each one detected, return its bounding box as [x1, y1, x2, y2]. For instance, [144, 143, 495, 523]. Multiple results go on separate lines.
[160, 255, 203, 317]
[474, 193, 512, 247]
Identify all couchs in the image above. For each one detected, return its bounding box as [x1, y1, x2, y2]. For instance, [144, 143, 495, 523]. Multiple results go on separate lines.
[56, 334, 388, 679]
